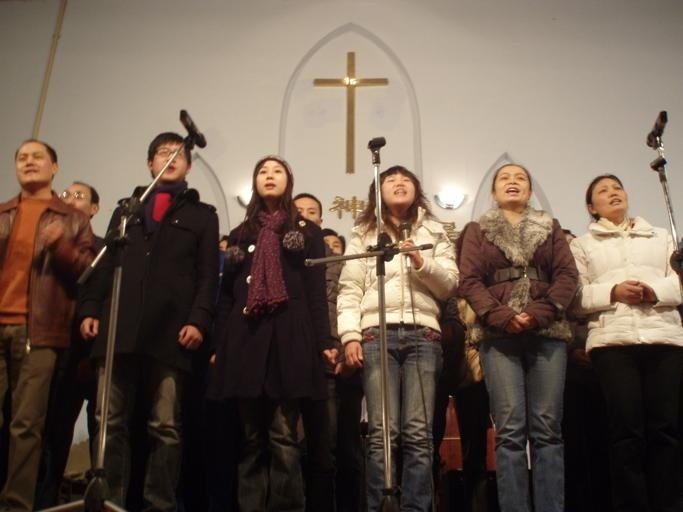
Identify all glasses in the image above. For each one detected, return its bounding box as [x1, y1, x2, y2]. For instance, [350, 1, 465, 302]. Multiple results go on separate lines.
[61, 191, 89, 201]
[157, 148, 186, 159]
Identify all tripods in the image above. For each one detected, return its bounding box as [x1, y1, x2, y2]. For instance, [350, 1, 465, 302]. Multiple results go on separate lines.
[38, 138, 192, 512]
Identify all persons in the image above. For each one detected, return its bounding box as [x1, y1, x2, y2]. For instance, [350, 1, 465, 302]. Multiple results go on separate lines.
[0, 131, 682, 510]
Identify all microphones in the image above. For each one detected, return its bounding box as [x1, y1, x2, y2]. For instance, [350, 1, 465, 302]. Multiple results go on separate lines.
[398, 220, 413, 269]
[646, 111, 668, 147]
[179, 109, 207, 149]
